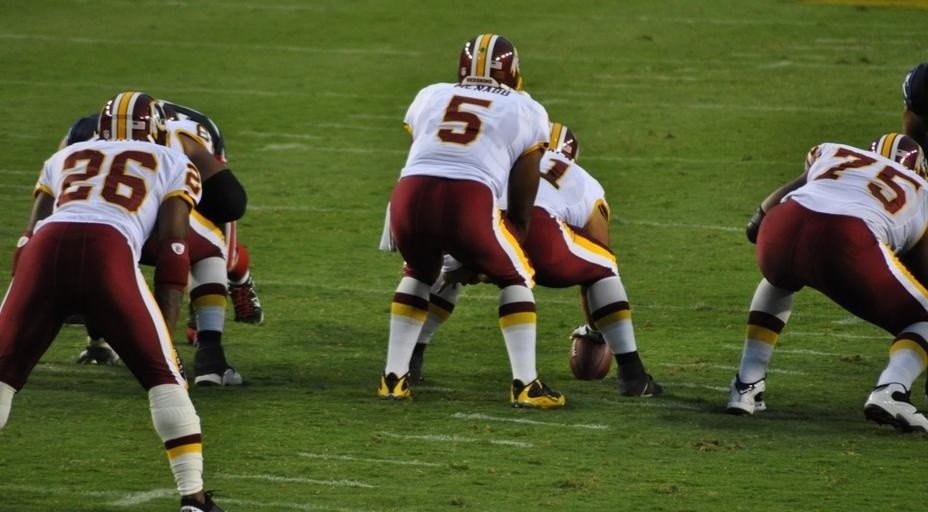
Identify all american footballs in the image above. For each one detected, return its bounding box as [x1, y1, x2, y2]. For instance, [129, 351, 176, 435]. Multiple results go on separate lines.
[570, 338, 612, 379]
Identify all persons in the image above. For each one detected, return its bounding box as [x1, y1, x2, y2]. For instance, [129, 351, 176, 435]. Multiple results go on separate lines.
[75, 106, 249, 390]
[375, 32, 569, 411]
[0, 91, 227, 512]
[901, 61, 928, 162]
[57, 96, 265, 348]
[406, 119, 664, 399]
[724, 132, 927, 437]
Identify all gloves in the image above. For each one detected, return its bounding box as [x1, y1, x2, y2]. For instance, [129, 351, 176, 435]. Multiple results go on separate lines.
[77, 336, 119, 365]
[569, 324, 605, 345]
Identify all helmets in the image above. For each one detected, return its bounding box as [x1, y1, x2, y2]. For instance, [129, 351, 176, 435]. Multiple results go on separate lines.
[869, 133, 928, 182]
[548, 122, 579, 162]
[458, 32, 523, 90]
[94, 91, 179, 148]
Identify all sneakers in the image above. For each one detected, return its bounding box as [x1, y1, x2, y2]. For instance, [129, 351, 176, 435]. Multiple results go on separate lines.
[227, 276, 264, 325]
[617, 368, 663, 398]
[377, 370, 424, 401]
[727, 373, 766, 415]
[510, 378, 566, 409]
[194, 366, 242, 386]
[864, 383, 928, 433]
[180, 490, 224, 512]
